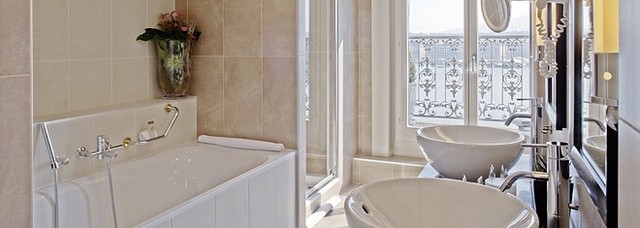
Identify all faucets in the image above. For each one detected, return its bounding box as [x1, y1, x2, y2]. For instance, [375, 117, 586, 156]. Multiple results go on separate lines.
[505, 97, 552, 140]
[95, 133, 118, 163]
[583, 117, 606, 133]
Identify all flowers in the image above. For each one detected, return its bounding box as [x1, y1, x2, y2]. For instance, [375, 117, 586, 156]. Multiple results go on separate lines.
[136, 10, 201, 41]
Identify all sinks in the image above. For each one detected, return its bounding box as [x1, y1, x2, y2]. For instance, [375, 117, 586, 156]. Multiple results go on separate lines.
[500, 141, 578, 221]
[417, 124, 525, 152]
[584, 133, 608, 153]
[344, 178, 540, 228]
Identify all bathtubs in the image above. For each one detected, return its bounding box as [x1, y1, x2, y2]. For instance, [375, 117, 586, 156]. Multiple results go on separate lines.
[31, 142, 294, 228]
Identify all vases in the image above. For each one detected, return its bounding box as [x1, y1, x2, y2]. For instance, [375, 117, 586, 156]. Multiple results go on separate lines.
[154, 39, 193, 98]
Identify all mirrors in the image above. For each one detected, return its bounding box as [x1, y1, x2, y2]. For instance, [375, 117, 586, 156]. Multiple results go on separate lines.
[566, 0, 620, 228]
[303, 1, 339, 222]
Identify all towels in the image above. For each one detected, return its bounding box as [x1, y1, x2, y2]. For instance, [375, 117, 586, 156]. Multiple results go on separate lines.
[197, 134, 284, 151]
